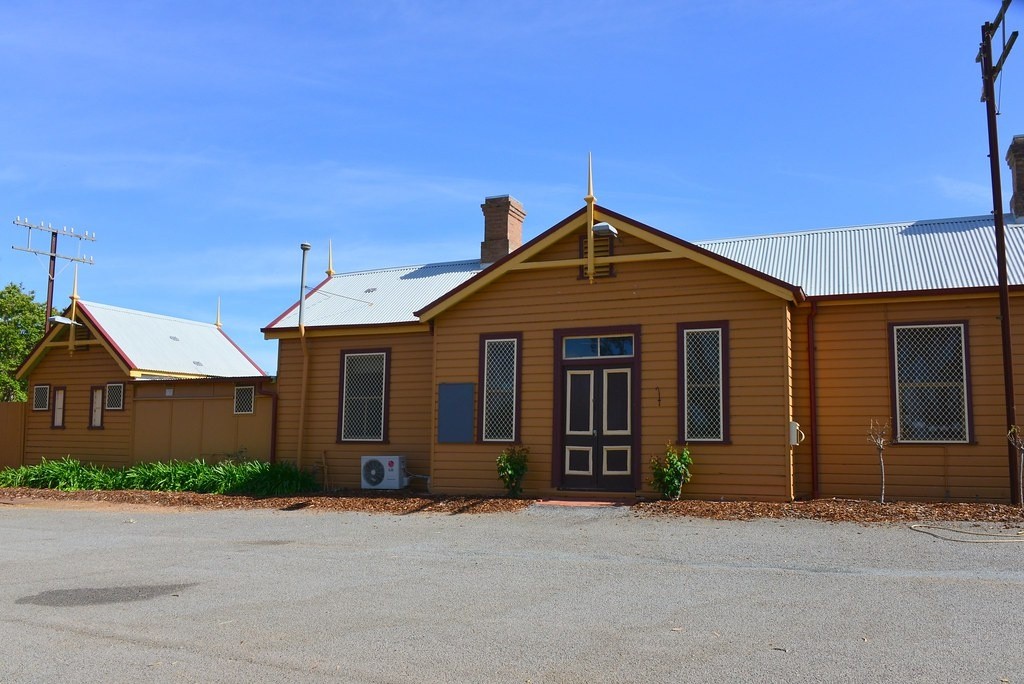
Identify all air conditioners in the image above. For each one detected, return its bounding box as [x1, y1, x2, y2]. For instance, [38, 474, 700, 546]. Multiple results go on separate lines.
[360, 455, 411, 491]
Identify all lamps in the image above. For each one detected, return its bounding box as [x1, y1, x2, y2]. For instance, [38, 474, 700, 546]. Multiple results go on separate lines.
[592, 220, 618, 238]
[48, 315, 84, 327]
